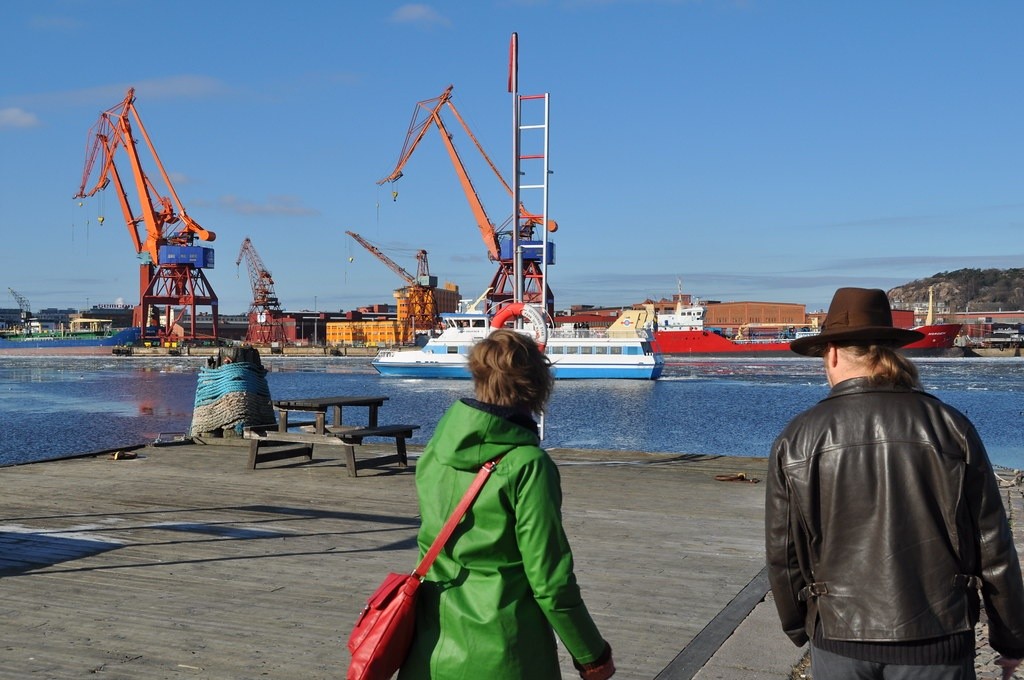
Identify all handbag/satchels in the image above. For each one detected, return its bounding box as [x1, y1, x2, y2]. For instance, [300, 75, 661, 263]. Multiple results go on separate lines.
[347, 570, 429, 679]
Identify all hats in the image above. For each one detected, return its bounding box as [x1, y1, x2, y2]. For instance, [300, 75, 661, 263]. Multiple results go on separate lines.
[790, 287, 925, 356]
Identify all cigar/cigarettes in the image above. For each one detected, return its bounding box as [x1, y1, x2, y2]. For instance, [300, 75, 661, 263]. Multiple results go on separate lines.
[1010, 672, 1019, 680]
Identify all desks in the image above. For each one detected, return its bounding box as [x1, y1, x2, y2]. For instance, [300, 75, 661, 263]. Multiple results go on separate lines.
[244, 396, 412, 478]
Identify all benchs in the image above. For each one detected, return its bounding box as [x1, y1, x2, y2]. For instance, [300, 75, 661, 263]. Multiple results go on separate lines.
[243, 420, 328, 431]
[327, 424, 421, 439]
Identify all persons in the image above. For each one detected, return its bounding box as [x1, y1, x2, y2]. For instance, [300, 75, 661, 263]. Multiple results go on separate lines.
[766, 286, 1024, 680]
[396, 330, 616, 680]
[586, 322, 590, 330]
[581, 322, 586, 329]
[574, 323, 578, 329]
[578, 322, 581, 328]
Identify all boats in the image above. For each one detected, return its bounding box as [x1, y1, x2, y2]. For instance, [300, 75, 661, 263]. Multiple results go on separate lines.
[648, 278, 967, 358]
[370, 313, 665, 379]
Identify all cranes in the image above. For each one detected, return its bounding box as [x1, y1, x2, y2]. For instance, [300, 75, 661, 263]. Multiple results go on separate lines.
[374, 82, 559, 265]
[235, 237, 293, 348]
[345, 229, 444, 349]
[72, 85, 216, 269]
[8, 286, 31, 337]
